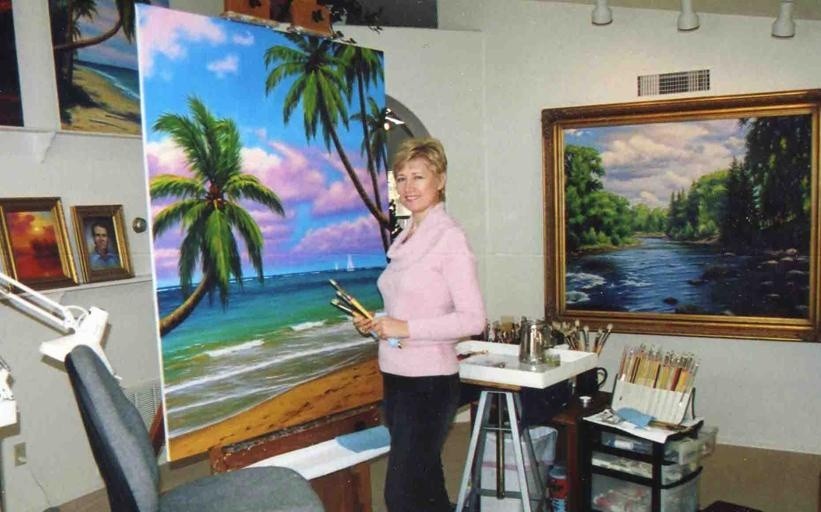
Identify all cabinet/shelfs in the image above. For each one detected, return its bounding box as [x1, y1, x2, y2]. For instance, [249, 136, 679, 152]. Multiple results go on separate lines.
[578, 419, 703, 512]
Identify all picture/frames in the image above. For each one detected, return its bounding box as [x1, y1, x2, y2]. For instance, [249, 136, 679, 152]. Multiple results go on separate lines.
[0, 197, 135, 294]
[542, 88, 821, 342]
[48, 0, 172, 137]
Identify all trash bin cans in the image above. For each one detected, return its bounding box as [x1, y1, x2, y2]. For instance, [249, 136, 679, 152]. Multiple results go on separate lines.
[481, 426, 558, 512]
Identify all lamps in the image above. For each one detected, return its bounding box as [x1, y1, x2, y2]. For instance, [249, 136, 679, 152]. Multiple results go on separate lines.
[678, 0, 699, 30]
[591, 0, 612, 24]
[0, 268, 118, 380]
[771, 2, 796, 37]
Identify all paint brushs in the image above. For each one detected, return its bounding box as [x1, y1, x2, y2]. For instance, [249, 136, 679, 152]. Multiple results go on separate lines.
[552, 320, 615, 359]
[327, 276, 403, 352]
[622, 343, 702, 392]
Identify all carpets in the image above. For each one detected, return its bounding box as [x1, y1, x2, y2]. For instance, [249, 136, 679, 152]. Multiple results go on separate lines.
[698, 499, 763, 512]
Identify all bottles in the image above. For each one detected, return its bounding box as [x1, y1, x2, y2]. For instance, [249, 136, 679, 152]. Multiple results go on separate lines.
[550, 461, 570, 512]
[518, 318, 549, 368]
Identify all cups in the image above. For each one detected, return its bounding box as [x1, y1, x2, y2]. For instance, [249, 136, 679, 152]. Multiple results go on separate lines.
[578, 366, 608, 396]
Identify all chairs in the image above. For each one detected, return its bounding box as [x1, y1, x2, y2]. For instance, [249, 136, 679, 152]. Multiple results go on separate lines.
[63, 343, 328, 512]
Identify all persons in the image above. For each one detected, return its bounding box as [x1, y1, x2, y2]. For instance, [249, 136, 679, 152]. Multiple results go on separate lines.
[86, 223, 121, 272]
[356, 138, 492, 511]
